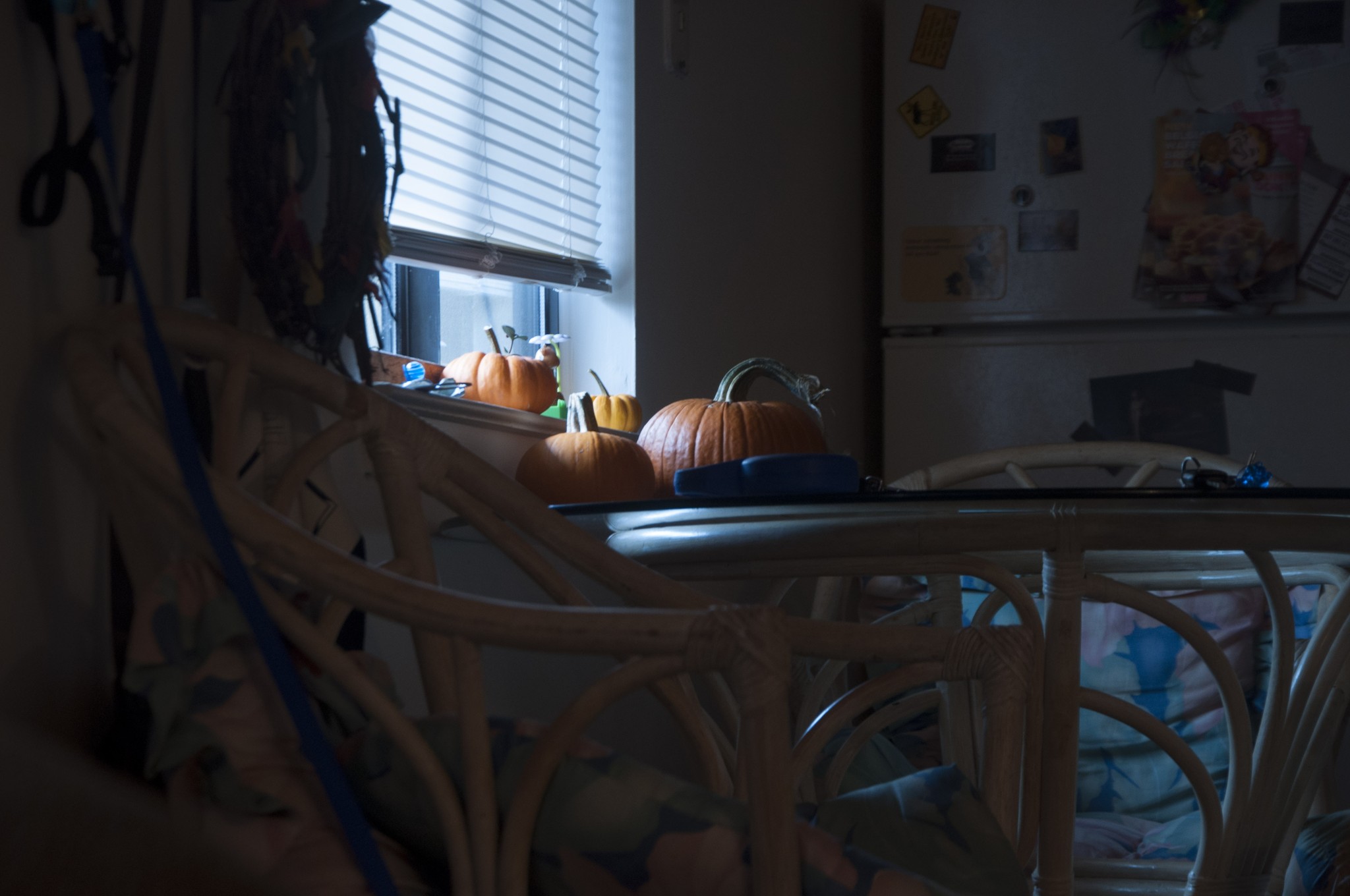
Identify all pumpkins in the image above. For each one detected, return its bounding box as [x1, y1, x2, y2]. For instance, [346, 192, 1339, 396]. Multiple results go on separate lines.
[635, 356, 828, 498]
[584, 370, 642, 431]
[515, 393, 654, 503]
[440, 325, 560, 414]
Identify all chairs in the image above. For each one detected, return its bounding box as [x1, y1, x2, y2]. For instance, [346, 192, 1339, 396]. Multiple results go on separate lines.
[794, 436, 1276, 893]
[61, 301, 1048, 894]
[604, 512, 1348, 896]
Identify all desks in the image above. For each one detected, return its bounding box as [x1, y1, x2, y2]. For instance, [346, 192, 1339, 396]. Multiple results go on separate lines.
[422, 478, 1350, 896]
